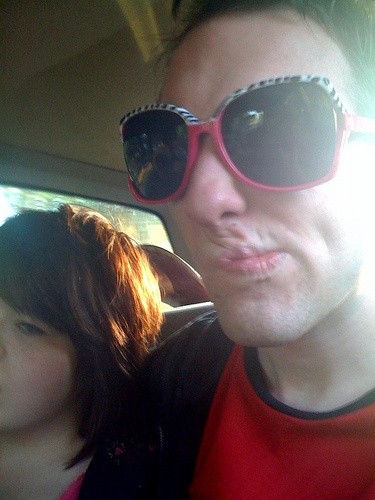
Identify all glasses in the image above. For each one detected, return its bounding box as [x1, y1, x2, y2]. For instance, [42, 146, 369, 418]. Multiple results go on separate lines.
[119, 76, 374, 206]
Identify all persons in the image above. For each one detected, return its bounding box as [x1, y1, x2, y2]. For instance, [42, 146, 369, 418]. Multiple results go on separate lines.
[119, 0, 375, 500]
[0, 201, 164, 500]
